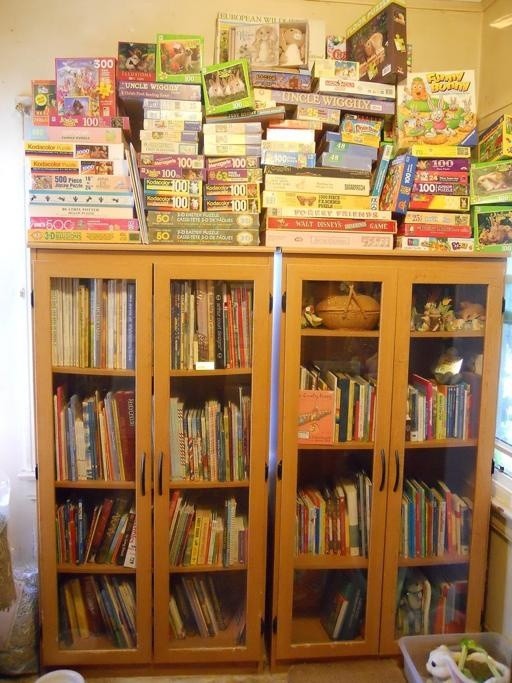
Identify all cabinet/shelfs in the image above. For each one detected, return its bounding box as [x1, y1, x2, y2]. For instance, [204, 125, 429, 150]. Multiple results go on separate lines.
[269, 248, 511, 670]
[26, 243, 276, 676]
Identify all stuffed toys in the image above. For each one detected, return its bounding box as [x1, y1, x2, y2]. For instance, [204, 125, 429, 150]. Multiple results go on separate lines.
[422, 638, 510, 683]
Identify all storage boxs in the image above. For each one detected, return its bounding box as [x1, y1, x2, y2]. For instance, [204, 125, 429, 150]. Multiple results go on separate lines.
[398, 633, 509, 682]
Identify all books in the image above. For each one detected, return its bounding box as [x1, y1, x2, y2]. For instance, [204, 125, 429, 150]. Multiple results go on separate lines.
[394, 568, 467, 635]
[50, 276, 135, 370]
[54, 494, 137, 569]
[168, 573, 247, 645]
[52, 382, 136, 482]
[294, 469, 369, 559]
[399, 478, 470, 559]
[319, 571, 365, 641]
[57, 573, 136, 649]
[170, 278, 252, 372]
[168, 489, 247, 568]
[402, 373, 475, 441]
[300, 361, 376, 444]
[169, 383, 252, 483]
